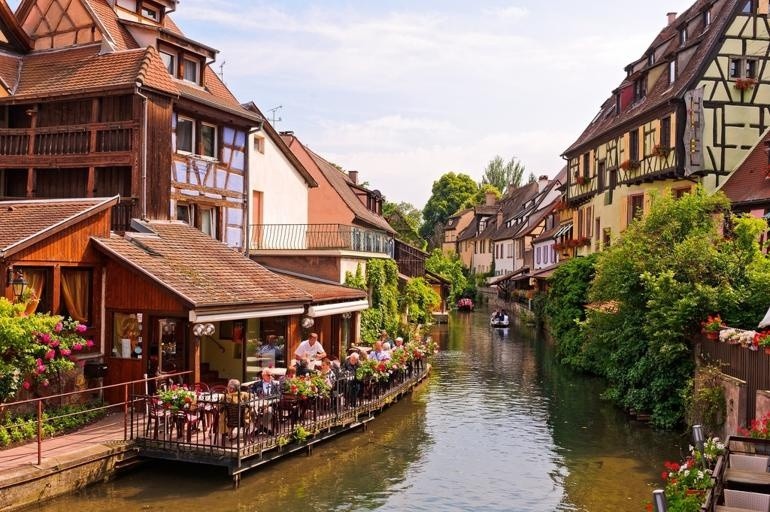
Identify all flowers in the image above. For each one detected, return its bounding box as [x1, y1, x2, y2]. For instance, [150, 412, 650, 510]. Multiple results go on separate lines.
[644, 436, 727, 512]
[736, 410, 770, 440]
[701, 313, 770, 355]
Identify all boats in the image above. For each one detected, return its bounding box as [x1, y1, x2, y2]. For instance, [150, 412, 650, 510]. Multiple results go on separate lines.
[491, 313, 509, 326]
[458, 299, 473, 311]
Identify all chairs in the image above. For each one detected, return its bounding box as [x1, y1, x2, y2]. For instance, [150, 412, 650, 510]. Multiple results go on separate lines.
[147, 343, 412, 446]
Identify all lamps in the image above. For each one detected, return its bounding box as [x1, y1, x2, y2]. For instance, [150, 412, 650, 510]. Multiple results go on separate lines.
[6, 264, 29, 304]
[343, 312, 352, 320]
[193, 323, 215, 336]
[301, 316, 314, 328]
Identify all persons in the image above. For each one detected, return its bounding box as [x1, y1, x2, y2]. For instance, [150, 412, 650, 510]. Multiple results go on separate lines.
[215, 379, 252, 436]
[494, 309, 508, 321]
[252, 368, 281, 435]
[284, 329, 404, 417]
[255, 335, 285, 359]
